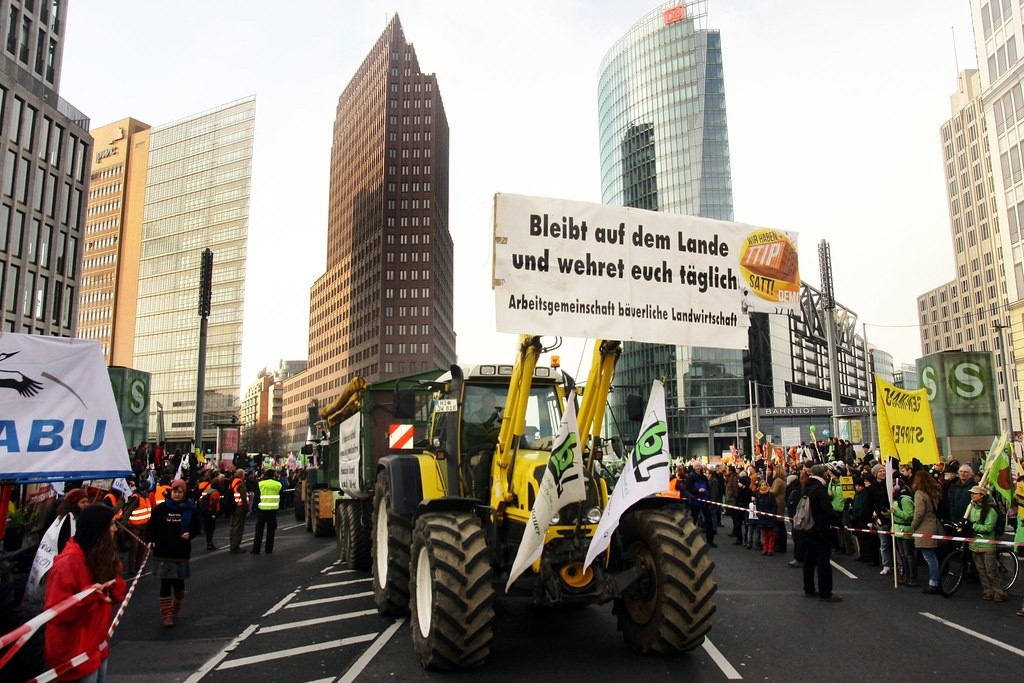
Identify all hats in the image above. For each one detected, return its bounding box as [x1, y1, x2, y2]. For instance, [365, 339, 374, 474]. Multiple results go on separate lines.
[943, 465, 958, 474]
[170, 479, 186, 495]
[831, 466, 843, 478]
[852, 464, 884, 487]
[738, 470, 747, 477]
[811, 465, 825, 477]
[740, 477, 749, 487]
[862, 444, 869, 449]
[65, 488, 89, 504]
[693, 462, 702, 469]
[265, 469, 275, 477]
[968, 486, 987, 496]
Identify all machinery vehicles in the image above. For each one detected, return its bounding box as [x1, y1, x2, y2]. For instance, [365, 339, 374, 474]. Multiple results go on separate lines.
[295, 332, 720, 673]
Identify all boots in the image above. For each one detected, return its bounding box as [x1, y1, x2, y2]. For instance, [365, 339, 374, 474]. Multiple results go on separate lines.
[160, 591, 185, 627]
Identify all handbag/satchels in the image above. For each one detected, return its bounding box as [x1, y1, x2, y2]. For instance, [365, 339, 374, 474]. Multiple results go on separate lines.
[748, 502, 759, 520]
[936, 518, 948, 545]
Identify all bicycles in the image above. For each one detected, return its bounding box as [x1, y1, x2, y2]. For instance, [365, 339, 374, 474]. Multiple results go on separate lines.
[939, 523, 1019, 597]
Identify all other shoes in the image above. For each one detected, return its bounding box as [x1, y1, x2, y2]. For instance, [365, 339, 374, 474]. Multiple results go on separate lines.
[207, 542, 260, 554]
[993, 593, 1009, 602]
[727, 532, 775, 555]
[709, 541, 718, 548]
[787, 559, 801, 568]
[833, 543, 941, 594]
[982, 589, 994, 600]
[819, 595, 842, 602]
[717, 522, 725, 528]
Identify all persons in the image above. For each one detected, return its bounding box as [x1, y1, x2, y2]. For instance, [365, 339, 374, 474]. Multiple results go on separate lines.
[468, 393, 503, 454]
[0, 443, 306, 683]
[671, 435, 1024, 617]
[313, 420, 327, 467]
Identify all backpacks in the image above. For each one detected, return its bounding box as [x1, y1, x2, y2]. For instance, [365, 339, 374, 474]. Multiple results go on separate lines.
[223, 479, 242, 516]
[192, 482, 217, 515]
[979, 504, 1006, 536]
[793, 483, 822, 531]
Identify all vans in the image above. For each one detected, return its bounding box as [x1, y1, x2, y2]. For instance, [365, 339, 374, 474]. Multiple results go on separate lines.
[205, 453, 272, 470]
